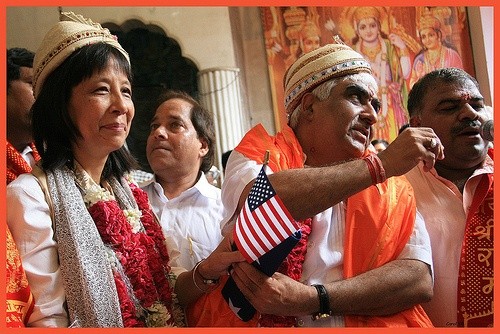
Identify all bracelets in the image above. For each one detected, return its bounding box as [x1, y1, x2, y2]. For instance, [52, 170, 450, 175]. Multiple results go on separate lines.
[193, 257, 224, 293]
[363, 153, 387, 184]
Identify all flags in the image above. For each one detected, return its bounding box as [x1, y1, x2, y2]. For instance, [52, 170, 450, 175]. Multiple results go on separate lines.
[236, 160, 302, 278]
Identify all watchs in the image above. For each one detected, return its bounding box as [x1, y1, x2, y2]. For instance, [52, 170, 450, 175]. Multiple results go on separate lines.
[196, 269, 220, 287]
[310, 284, 334, 321]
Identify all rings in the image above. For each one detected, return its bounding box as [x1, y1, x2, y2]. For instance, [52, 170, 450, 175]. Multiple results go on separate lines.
[429, 136, 437, 150]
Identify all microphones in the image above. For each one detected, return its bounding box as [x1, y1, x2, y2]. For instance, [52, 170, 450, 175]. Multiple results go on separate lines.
[479, 119, 494, 142]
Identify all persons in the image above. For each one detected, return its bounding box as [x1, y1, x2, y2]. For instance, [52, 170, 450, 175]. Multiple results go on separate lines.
[394, 67, 494, 328]
[137, 91, 225, 289]
[218, 36, 436, 328]
[6, 45, 37, 168]
[5, 12, 246, 328]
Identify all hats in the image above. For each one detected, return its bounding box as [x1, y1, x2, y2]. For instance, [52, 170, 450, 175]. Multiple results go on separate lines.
[283, 44, 372, 115]
[32, 11, 131, 100]
